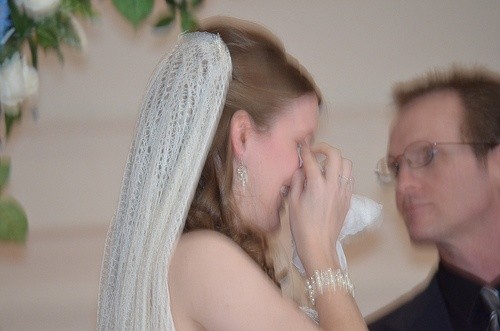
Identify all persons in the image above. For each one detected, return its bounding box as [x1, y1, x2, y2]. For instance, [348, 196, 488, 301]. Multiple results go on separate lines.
[362, 67, 499, 331]
[98, 18, 373, 331]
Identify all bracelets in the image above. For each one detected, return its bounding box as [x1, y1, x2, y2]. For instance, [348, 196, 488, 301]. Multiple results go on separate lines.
[305, 267, 356, 311]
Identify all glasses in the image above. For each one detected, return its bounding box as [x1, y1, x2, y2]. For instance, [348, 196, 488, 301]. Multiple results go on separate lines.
[374, 137, 494, 184]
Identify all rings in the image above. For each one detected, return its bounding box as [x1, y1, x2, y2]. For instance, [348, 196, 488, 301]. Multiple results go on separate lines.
[342, 175, 353, 184]
